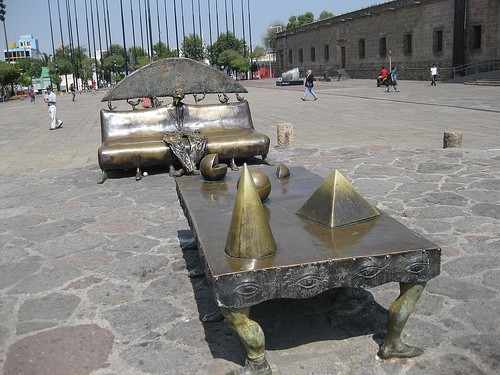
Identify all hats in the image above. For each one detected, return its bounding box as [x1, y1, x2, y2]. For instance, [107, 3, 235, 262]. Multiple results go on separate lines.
[46, 85, 52, 90]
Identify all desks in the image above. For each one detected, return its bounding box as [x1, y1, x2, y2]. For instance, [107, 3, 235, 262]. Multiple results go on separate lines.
[174, 167, 442, 375]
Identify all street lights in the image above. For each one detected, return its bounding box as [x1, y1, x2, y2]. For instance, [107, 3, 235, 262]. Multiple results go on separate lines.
[114, 58, 117, 83]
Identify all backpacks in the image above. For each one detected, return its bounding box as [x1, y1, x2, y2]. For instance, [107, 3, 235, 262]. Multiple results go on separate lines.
[387, 71, 392, 80]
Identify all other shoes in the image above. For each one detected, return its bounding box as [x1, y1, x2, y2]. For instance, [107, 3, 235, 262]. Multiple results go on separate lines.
[313, 97, 318, 101]
[300, 97, 305, 101]
[58, 122, 63, 128]
[49, 128, 54, 130]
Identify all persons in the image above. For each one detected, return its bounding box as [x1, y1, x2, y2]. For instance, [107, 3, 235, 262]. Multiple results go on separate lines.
[70, 83, 75, 102]
[378, 66, 400, 93]
[430, 64, 440, 86]
[29, 85, 36, 103]
[92, 80, 96, 90]
[301, 69, 318, 101]
[43, 85, 64, 130]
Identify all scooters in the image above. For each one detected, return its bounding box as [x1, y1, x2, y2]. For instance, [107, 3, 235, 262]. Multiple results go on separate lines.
[376, 74, 388, 87]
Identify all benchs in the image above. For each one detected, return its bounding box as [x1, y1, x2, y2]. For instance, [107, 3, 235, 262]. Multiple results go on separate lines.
[97, 100, 276, 184]
